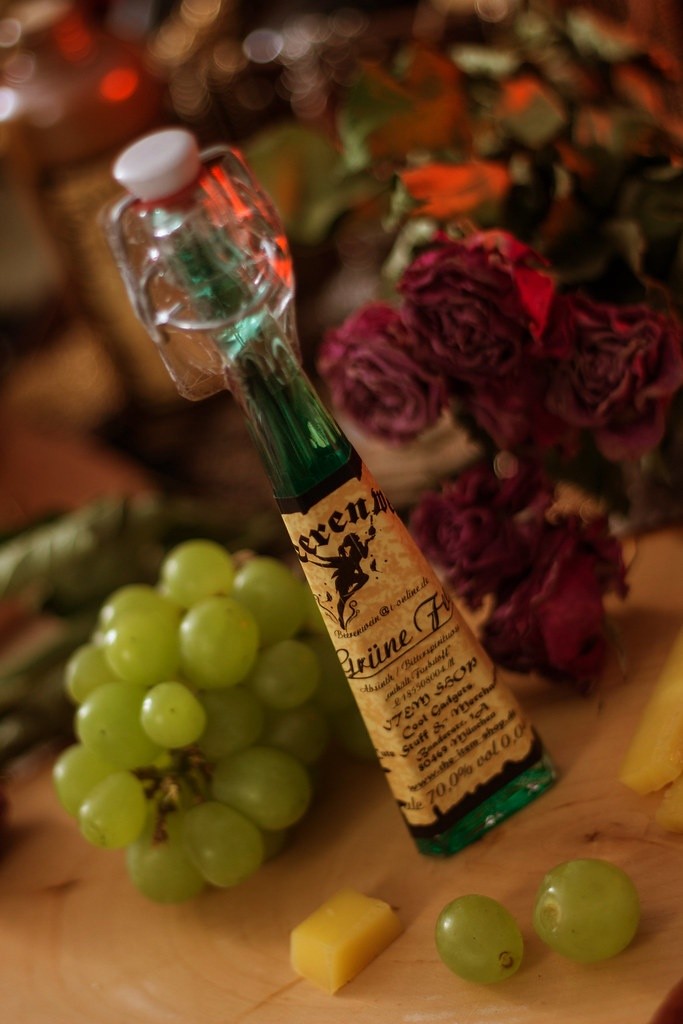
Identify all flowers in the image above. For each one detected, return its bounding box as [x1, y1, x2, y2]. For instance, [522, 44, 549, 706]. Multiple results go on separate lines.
[324, 213, 683, 684]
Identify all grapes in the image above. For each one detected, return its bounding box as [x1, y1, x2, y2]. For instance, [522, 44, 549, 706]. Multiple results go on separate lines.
[533, 859, 642, 960]
[435, 894, 525, 984]
[54, 537, 374, 902]
